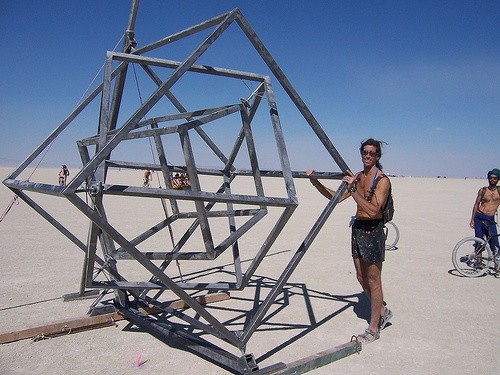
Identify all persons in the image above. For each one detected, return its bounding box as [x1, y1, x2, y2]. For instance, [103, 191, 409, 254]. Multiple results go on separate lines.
[143, 170, 153, 186]
[306, 137, 394, 345]
[58, 165, 70, 187]
[469, 168, 500, 278]
[171, 173, 191, 190]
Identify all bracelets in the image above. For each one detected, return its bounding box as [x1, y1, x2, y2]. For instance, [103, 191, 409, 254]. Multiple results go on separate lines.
[313, 180, 320, 187]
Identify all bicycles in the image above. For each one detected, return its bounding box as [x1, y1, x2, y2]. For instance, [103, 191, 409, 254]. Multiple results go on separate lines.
[142, 179, 151, 190]
[58, 174, 69, 190]
[350, 215, 400, 251]
[452, 221, 499, 278]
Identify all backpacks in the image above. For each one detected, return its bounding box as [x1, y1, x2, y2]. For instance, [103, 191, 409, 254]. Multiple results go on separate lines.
[350, 171, 396, 224]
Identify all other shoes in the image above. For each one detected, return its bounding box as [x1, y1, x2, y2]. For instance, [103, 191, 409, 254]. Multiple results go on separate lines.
[495, 270, 500, 278]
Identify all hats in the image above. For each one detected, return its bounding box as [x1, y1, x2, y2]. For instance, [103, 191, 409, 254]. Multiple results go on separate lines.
[488, 168, 500, 178]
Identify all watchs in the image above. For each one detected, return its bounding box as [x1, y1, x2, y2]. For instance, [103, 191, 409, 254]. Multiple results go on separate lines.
[348, 187, 357, 193]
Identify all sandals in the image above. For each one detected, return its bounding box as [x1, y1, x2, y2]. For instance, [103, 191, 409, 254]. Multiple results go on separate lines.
[378, 307, 393, 330]
[356, 329, 381, 344]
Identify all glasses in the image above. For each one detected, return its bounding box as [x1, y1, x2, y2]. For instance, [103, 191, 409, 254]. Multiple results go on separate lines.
[362, 150, 379, 157]
